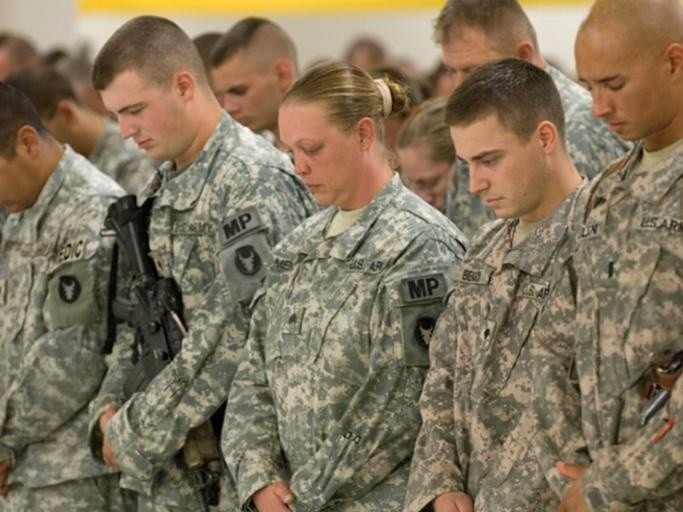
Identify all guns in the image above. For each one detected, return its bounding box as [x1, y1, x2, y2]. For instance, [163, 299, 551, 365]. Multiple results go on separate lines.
[100, 188, 224, 512]
